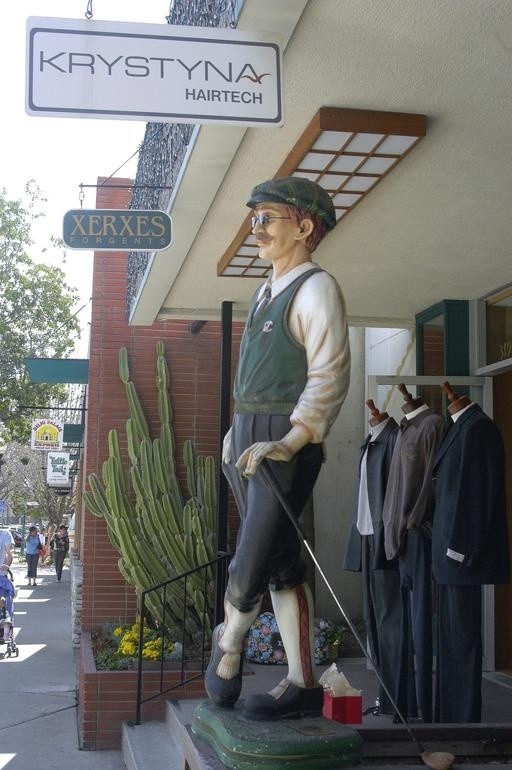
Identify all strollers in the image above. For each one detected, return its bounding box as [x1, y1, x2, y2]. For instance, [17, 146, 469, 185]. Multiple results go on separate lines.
[0, 568, 22, 659]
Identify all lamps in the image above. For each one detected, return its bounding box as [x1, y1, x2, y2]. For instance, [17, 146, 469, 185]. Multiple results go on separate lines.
[214, 106, 426, 278]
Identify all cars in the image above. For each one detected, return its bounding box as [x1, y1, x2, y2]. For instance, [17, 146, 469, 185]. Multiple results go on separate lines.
[0, 523, 33, 548]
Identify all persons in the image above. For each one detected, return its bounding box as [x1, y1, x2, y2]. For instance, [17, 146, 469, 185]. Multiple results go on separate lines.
[0, 529, 14, 643]
[203, 176, 351, 721]
[24, 524, 69, 586]
